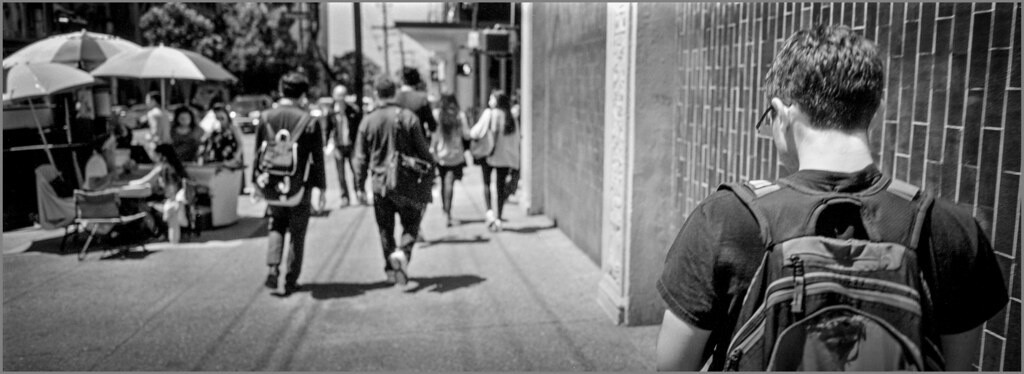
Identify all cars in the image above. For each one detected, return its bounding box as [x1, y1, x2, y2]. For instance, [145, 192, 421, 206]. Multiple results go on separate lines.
[117, 102, 185, 131]
[309, 97, 379, 127]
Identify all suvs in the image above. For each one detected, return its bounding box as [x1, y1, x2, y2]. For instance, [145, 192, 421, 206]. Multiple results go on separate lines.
[232, 95, 274, 134]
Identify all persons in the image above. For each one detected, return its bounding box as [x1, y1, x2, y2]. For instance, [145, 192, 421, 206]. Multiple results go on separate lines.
[467, 88, 520, 231]
[428, 87, 473, 225]
[352, 76, 438, 284]
[248, 71, 328, 293]
[294, 83, 339, 217]
[80, 89, 247, 240]
[323, 83, 370, 210]
[655, 25, 1010, 374]
[386, 66, 439, 250]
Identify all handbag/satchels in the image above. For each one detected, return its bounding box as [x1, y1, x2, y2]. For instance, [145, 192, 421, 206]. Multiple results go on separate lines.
[470, 129, 494, 160]
[387, 151, 434, 203]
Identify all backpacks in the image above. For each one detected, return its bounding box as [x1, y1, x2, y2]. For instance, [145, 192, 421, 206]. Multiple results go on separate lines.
[255, 110, 312, 201]
[699, 178, 934, 372]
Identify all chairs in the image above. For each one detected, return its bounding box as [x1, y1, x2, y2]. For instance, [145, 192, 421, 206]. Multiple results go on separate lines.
[147, 178, 203, 242]
[36, 165, 82, 250]
[73, 188, 148, 260]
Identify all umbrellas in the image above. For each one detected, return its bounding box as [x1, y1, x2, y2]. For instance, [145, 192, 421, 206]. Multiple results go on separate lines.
[3, 61, 110, 183]
[88, 42, 238, 113]
[4, 28, 147, 75]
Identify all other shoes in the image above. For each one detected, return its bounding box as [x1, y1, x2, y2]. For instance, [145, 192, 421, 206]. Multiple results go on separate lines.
[264, 273, 277, 289]
[389, 249, 409, 286]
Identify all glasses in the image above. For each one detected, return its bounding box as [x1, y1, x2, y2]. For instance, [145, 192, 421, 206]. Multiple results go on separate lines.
[755, 106, 775, 140]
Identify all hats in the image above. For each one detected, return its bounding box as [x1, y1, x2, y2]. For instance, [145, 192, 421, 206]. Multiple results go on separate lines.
[282, 72, 305, 98]
[404, 66, 420, 85]
